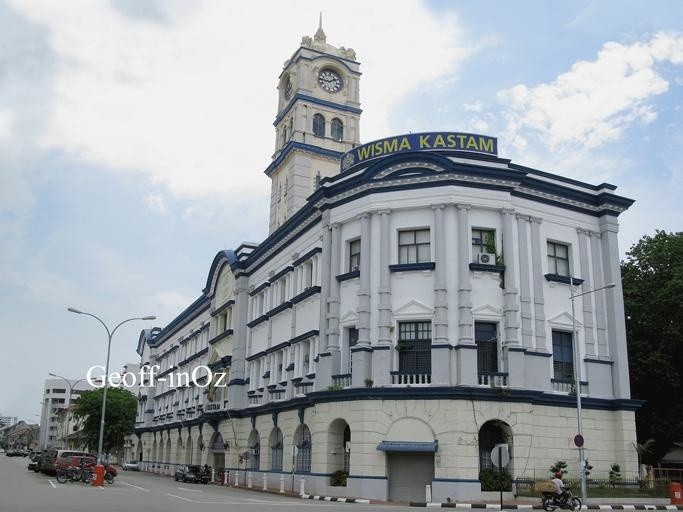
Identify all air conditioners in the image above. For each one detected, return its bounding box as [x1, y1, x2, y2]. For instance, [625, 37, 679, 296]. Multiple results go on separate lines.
[477, 253, 496, 265]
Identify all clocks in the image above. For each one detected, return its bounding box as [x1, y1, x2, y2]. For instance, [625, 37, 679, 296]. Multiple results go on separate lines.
[284, 79, 293, 100]
[318, 69, 344, 94]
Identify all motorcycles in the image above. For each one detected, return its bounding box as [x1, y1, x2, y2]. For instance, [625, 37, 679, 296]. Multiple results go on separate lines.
[56, 460, 114, 484]
[540, 481, 582, 512]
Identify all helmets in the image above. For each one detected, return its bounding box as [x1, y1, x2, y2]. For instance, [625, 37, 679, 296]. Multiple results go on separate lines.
[555, 472, 561, 479]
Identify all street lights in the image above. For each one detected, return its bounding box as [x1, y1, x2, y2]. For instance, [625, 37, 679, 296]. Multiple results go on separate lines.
[68, 307, 156, 487]
[568, 277, 616, 504]
[48, 373, 96, 449]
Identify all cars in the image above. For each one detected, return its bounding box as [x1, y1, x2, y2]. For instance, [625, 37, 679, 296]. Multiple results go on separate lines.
[28, 450, 118, 480]
[121, 461, 139, 471]
[6, 449, 31, 458]
[174, 464, 208, 484]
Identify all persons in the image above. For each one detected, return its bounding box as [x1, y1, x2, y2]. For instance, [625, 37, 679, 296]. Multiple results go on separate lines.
[551, 471, 573, 508]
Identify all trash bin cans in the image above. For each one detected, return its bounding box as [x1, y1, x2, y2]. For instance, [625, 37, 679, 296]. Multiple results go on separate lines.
[670, 482, 683, 503]
[92, 464, 105, 485]
